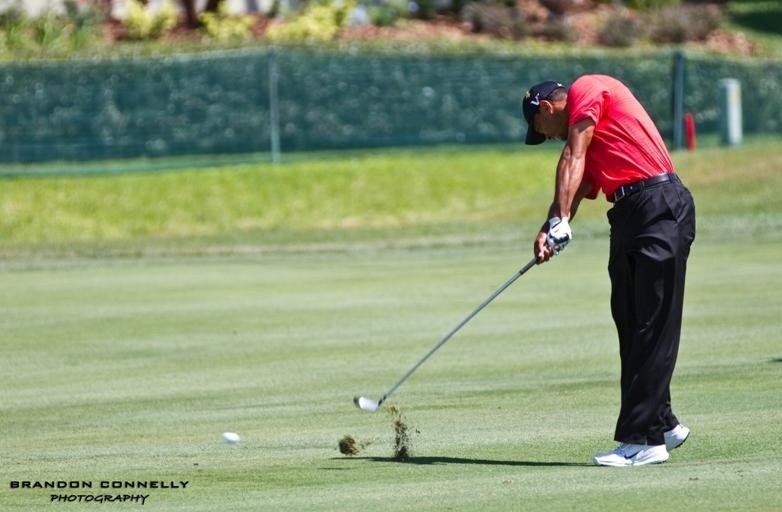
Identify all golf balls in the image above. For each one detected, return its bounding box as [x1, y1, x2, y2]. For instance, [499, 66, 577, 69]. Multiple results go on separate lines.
[223, 430, 240, 446]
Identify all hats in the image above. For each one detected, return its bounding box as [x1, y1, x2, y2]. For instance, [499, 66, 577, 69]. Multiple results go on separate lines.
[523, 81, 566, 144]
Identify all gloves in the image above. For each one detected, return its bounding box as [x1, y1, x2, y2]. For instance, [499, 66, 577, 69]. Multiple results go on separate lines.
[547, 215, 573, 255]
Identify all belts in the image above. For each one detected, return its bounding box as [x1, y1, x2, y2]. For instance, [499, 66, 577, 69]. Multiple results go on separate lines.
[613, 172, 679, 202]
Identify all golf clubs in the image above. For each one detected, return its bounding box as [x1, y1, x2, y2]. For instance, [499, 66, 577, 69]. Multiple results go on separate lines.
[353, 253, 538, 413]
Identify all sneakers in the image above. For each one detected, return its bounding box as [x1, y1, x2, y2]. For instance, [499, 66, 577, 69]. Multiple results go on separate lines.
[593, 422, 689, 467]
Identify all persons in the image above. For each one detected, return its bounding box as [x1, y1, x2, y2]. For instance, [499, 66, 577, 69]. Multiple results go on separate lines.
[521, 72, 697, 470]
